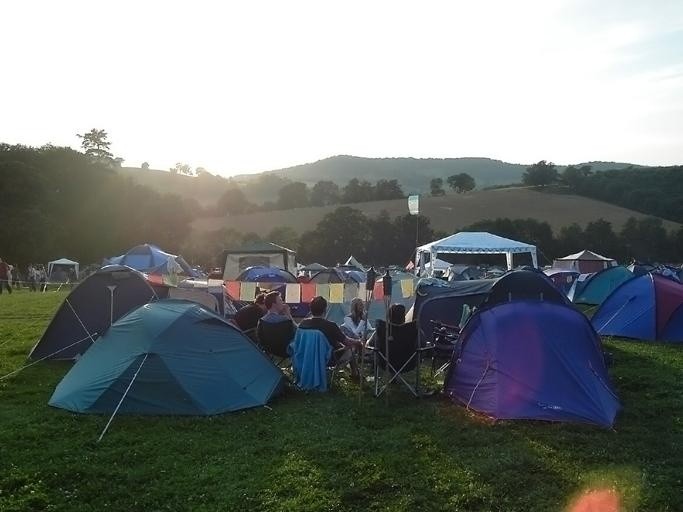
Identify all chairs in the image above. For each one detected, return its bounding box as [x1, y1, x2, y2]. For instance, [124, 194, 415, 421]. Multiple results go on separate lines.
[257, 317, 460, 399]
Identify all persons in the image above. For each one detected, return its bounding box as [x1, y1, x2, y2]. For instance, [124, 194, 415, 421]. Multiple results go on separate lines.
[343, 298, 373, 337]
[234, 292, 268, 329]
[365, 301, 428, 351]
[298, 292, 362, 380]
[0, 256, 49, 295]
[257, 289, 297, 335]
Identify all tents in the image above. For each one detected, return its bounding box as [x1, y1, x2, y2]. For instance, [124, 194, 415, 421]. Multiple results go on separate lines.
[105, 242, 419, 318]
[44, 296, 289, 419]
[410, 248, 682, 436]
[24, 261, 158, 363]
